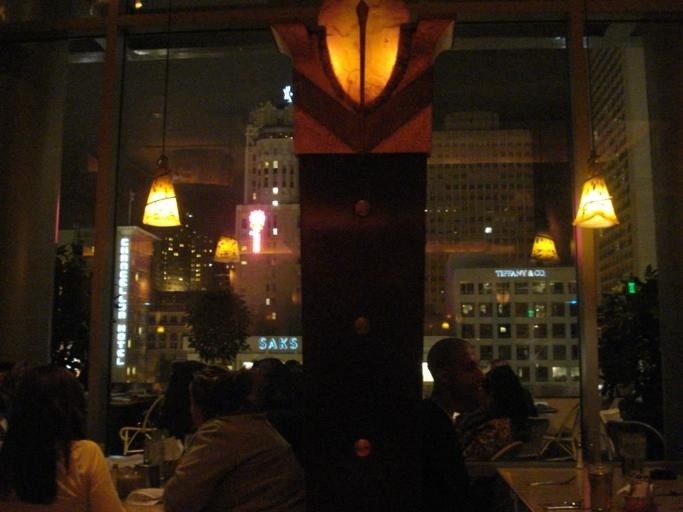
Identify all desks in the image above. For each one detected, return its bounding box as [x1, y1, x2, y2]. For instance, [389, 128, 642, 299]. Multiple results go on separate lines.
[499, 466, 683, 511]
[104, 390, 168, 512]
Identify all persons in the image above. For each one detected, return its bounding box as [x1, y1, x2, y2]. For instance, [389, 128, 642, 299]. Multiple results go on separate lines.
[1, 359, 125, 512]
[394, 335, 535, 511]
[143, 357, 315, 511]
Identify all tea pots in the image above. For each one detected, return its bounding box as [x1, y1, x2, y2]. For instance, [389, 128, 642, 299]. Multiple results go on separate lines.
[629, 475, 655, 496]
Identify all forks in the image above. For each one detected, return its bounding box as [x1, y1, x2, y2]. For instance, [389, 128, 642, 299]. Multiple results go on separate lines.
[527, 475, 577, 487]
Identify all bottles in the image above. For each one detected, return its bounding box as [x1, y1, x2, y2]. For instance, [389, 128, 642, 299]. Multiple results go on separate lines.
[135, 459, 160, 488]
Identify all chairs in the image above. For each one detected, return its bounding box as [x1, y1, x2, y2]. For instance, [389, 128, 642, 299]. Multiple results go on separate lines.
[492, 402, 666, 462]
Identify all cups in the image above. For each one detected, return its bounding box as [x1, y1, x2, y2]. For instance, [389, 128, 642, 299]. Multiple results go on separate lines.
[588, 465, 613, 511]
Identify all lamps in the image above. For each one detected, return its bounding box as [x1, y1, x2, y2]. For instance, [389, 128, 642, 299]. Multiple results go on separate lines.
[530, 78, 561, 264]
[214, 31, 242, 264]
[571, 1, 620, 230]
[141, 0, 180, 230]
